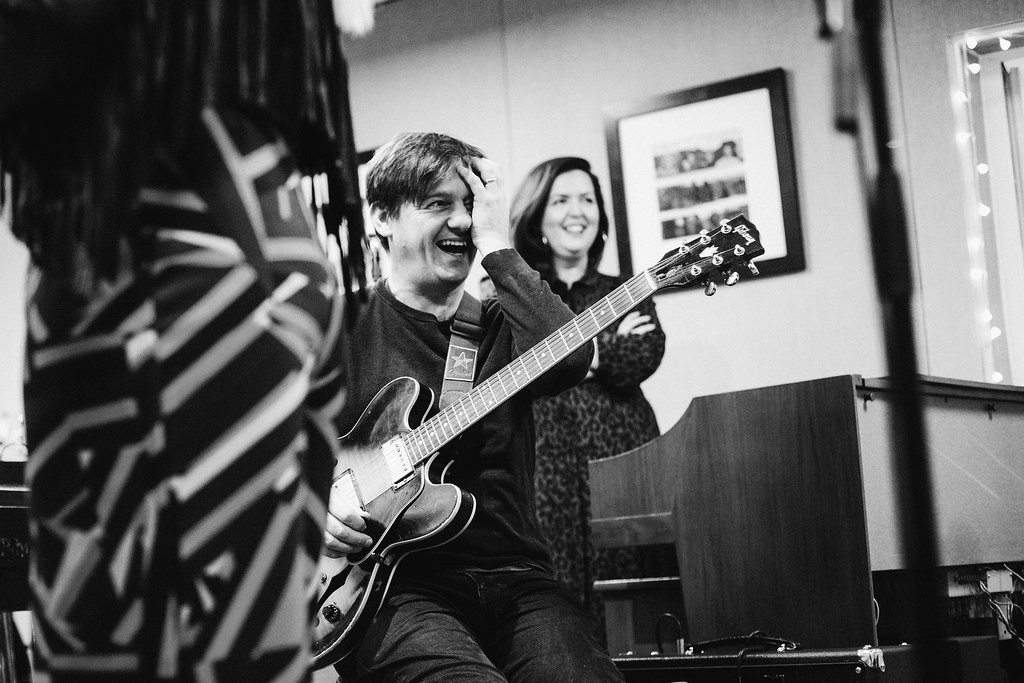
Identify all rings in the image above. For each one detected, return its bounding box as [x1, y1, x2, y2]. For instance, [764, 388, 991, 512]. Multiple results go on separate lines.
[485, 178, 496, 184]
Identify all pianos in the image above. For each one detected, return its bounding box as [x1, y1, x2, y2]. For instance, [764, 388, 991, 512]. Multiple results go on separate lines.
[582, 371, 1022, 683]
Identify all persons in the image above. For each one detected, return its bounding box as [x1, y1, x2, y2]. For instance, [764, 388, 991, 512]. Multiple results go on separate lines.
[478, 157, 678, 650]
[0, 0, 626, 683]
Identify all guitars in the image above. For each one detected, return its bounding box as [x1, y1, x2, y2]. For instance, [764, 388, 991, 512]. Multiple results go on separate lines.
[303, 210, 767, 675]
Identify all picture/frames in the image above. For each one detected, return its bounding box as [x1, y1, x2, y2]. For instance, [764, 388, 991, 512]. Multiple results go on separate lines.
[601, 66, 809, 293]
